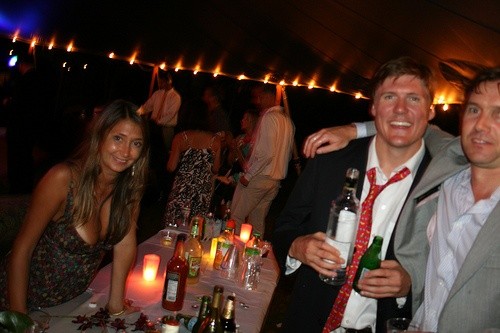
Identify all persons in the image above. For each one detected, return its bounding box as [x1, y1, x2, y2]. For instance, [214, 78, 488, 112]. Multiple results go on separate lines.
[137, 70, 181, 128]
[0, 100, 149, 333]
[161, 98, 221, 228]
[264, 62, 435, 333]
[218, 109, 258, 198]
[231, 85, 297, 234]
[304, 69, 500, 333]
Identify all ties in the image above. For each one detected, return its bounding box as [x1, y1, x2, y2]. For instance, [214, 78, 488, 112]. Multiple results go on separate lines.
[155, 92, 170, 120]
[242, 108, 270, 172]
[322, 166, 410, 333]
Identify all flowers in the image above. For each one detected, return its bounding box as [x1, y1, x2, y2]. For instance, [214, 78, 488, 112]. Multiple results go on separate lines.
[38, 308, 157, 333]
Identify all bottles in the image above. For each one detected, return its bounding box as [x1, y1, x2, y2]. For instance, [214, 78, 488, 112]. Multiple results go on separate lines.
[162, 233, 190, 311]
[204, 207, 261, 291]
[176, 285, 236, 333]
[353, 236, 383, 295]
[318, 168, 361, 286]
[186, 218, 202, 278]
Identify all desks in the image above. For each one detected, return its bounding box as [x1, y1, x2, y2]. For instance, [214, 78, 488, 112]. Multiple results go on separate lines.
[27, 227, 280, 333]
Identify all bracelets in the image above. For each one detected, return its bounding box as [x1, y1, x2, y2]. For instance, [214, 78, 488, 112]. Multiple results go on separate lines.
[105, 303, 127, 315]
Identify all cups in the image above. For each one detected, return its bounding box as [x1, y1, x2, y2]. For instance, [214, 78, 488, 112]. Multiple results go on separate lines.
[240, 223, 252, 243]
[261, 242, 271, 257]
[160, 230, 176, 247]
[0, 310, 35, 333]
[161, 323, 180, 333]
[386, 318, 422, 333]
[25, 307, 50, 333]
[142, 254, 160, 282]
[209, 238, 225, 259]
